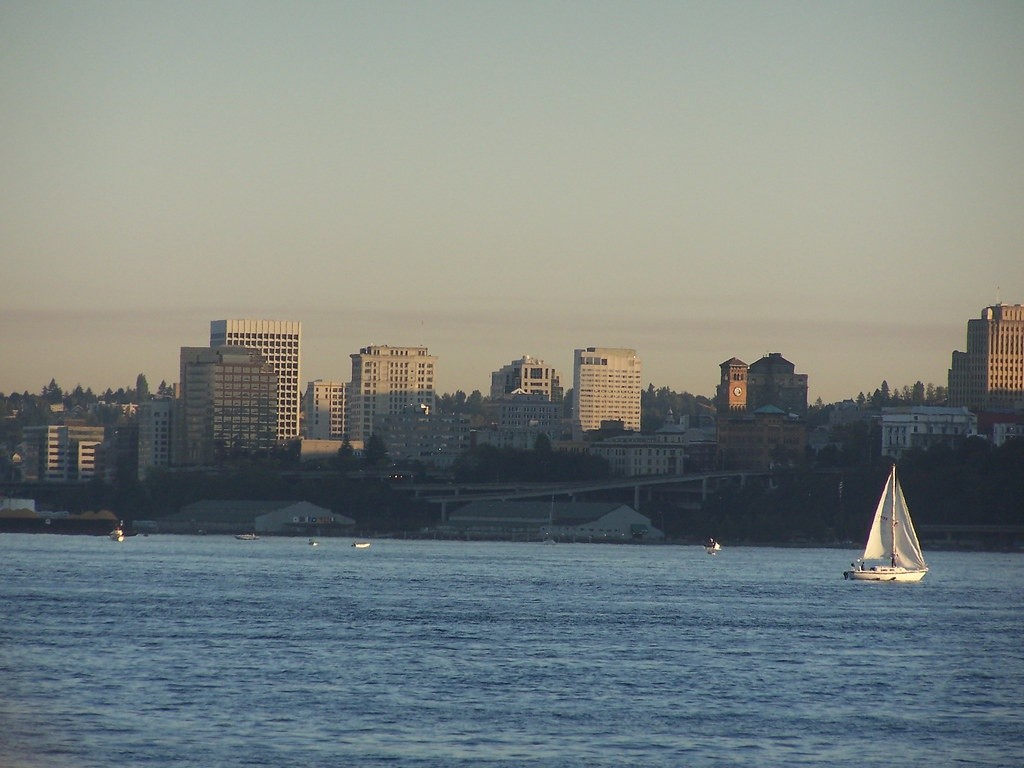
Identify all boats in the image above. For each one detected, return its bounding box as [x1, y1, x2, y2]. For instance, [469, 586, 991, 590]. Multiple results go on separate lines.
[352, 542, 370, 550]
[703, 539, 723, 554]
[234, 533, 261, 541]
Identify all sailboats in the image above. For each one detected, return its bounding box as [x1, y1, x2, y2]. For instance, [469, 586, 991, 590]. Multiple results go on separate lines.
[844, 463, 930, 581]
